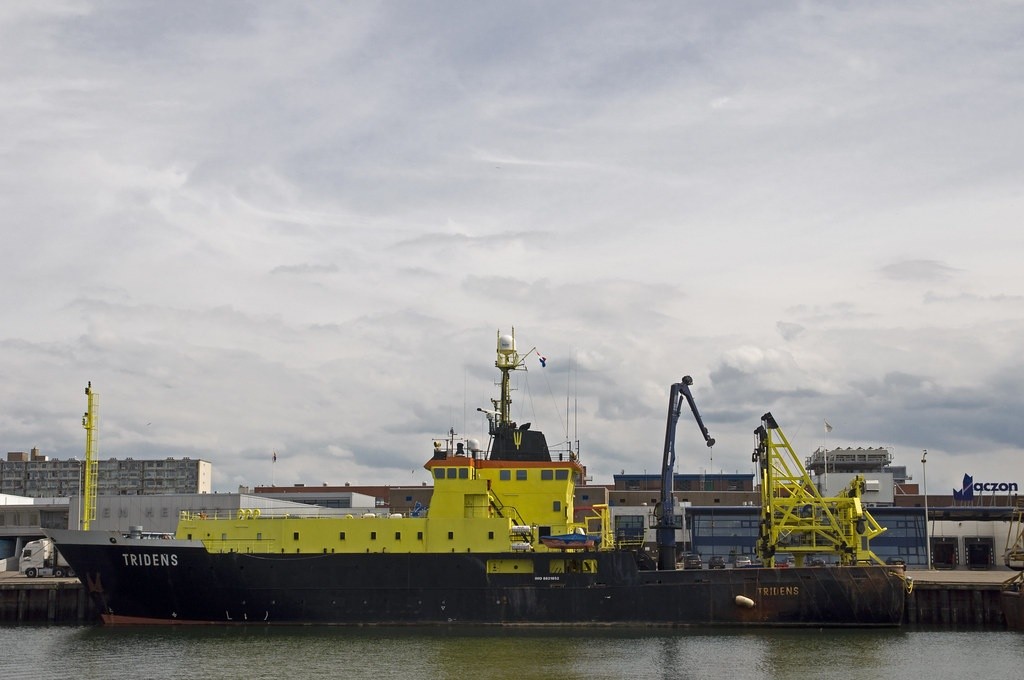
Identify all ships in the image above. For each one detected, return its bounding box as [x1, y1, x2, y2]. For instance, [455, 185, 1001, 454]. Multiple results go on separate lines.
[39, 324, 916, 626]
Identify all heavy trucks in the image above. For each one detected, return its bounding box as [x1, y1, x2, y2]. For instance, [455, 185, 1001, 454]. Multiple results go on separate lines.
[18, 538, 76, 578]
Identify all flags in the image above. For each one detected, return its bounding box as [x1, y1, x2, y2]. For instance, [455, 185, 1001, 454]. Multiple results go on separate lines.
[824, 419, 832, 432]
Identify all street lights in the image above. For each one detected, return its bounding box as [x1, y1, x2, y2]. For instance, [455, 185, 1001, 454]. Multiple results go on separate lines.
[921, 448, 931, 571]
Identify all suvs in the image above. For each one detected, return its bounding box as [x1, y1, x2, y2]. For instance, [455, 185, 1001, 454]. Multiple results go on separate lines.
[683, 554, 702, 569]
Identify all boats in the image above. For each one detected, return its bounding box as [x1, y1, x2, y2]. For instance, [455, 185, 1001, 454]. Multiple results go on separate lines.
[539, 534, 602, 549]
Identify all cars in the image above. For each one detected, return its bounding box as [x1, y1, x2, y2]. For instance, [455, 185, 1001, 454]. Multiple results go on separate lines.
[733, 556, 752, 568]
[806, 556, 825, 566]
[885, 557, 906, 571]
[709, 556, 725, 569]
